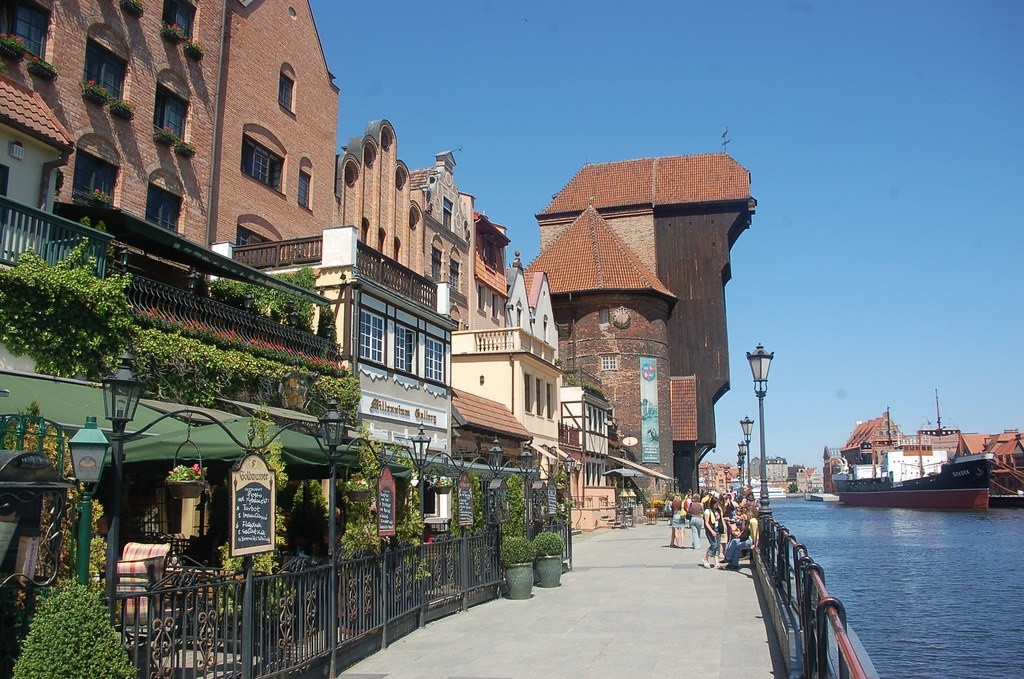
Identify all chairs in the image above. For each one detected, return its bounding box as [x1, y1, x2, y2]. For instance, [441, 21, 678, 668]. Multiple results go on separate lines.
[117, 542, 193, 659]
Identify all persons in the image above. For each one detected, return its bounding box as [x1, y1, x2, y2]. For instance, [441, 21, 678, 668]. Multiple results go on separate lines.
[664, 484, 759, 571]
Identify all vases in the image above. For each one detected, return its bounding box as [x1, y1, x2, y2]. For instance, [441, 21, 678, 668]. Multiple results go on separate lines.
[346, 490, 371, 502]
[82, 88, 108, 108]
[168, 482, 205, 499]
[174, 145, 196, 160]
[432, 486, 453, 494]
[649, 504, 666, 512]
[160, 29, 181, 45]
[184, 44, 204, 62]
[119, 0, 143, 19]
[27, 62, 58, 81]
[154, 133, 175, 148]
[0, 42, 24, 65]
[109, 104, 134, 120]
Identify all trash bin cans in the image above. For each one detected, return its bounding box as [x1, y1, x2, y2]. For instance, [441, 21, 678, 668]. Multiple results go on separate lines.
[624, 507, 633, 526]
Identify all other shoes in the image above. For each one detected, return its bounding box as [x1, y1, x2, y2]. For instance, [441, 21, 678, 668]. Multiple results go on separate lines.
[714, 564, 723, 568]
[702, 559, 710, 568]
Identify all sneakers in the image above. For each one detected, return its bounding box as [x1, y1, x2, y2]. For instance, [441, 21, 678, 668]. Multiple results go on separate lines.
[723, 564, 734, 569]
[719, 558, 732, 563]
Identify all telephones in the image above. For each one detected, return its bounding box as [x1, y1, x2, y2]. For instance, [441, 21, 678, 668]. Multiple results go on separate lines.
[457, 473, 474, 525]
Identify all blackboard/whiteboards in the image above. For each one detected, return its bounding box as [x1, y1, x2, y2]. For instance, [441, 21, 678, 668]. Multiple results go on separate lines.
[227, 452, 276, 557]
[548, 483, 558, 514]
[377, 465, 395, 536]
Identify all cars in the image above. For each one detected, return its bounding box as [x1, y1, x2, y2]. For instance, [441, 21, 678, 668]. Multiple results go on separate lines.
[729, 480, 740, 488]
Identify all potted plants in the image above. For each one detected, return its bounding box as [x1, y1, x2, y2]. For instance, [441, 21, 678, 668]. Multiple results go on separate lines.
[500, 536, 538, 600]
[583, 381, 610, 402]
[673, 492, 685, 511]
[532, 532, 564, 587]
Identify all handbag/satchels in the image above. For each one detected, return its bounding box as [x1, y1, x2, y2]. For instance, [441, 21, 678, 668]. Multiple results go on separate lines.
[717, 520, 725, 534]
[740, 529, 750, 541]
[686, 511, 692, 520]
[680, 509, 687, 518]
[664, 510, 672, 518]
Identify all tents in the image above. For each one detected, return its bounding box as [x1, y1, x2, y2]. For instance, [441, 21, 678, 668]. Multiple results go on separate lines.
[103, 418, 413, 652]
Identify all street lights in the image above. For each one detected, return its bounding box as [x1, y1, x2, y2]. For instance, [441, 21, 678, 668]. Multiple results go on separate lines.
[740, 415, 755, 486]
[724, 470, 729, 493]
[488, 436, 504, 579]
[562, 452, 575, 558]
[746, 343, 775, 510]
[66, 416, 111, 585]
[738, 441, 747, 485]
[411, 423, 431, 604]
[519, 444, 533, 540]
[100, 345, 146, 627]
[317, 396, 348, 648]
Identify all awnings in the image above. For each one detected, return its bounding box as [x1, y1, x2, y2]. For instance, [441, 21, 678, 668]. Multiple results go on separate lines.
[602, 468, 646, 478]
[0, 375, 354, 440]
[529, 443, 582, 471]
[609, 456, 674, 480]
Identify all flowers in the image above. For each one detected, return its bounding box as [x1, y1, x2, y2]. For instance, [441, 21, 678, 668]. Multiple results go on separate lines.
[32, 56, 54, 71]
[80, 80, 108, 97]
[0, 32, 26, 50]
[109, 95, 138, 113]
[345, 472, 369, 489]
[155, 126, 177, 139]
[648, 499, 665, 504]
[177, 139, 197, 150]
[433, 477, 453, 487]
[165, 463, 207, 481]
[130, 0, 148, 10]
[161, 20, 183, 40]
[186, 37, 205, 55]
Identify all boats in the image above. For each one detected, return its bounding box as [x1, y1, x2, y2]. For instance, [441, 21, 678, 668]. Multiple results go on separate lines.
[833, 388, 995, 510]
[751, 482, 786, 499]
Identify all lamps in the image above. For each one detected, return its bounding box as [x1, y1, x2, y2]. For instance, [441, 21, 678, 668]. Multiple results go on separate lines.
[325, 321, 336, 339]
[480, 375, 484, 385]
[186, 267, 199, 294]
[619, 488, 629, 506]
[627, 488, 637, 505]
[289, 311, 300, 329]
[118, 244, 132, 269]
[243, 292, 255, 313]
